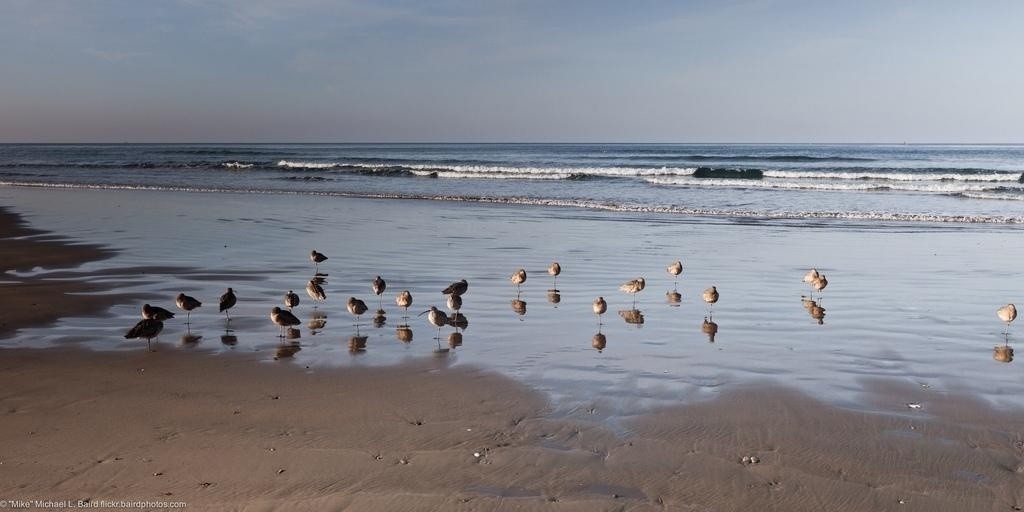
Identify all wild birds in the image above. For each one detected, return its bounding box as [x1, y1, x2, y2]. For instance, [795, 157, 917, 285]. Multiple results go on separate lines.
[617, 301, 644, 323]
[801, 268, 828, 298]
[592, 324, 606, 354]
[993, 334, 1014, 362]
[618, 277, 645, 302]
[996, 304, 1017, 329]
[510, 261, 561, 293]
[702, 287, 719, 311]
[699, 312, 718, 342]
[664, 282, 681, 309]
[666, 261, 682, 283]
[802, 291, 826, 325]
[511, 279, 562, 315]
[124, 247, 468, 351]
[592, 296, 607, 324]
